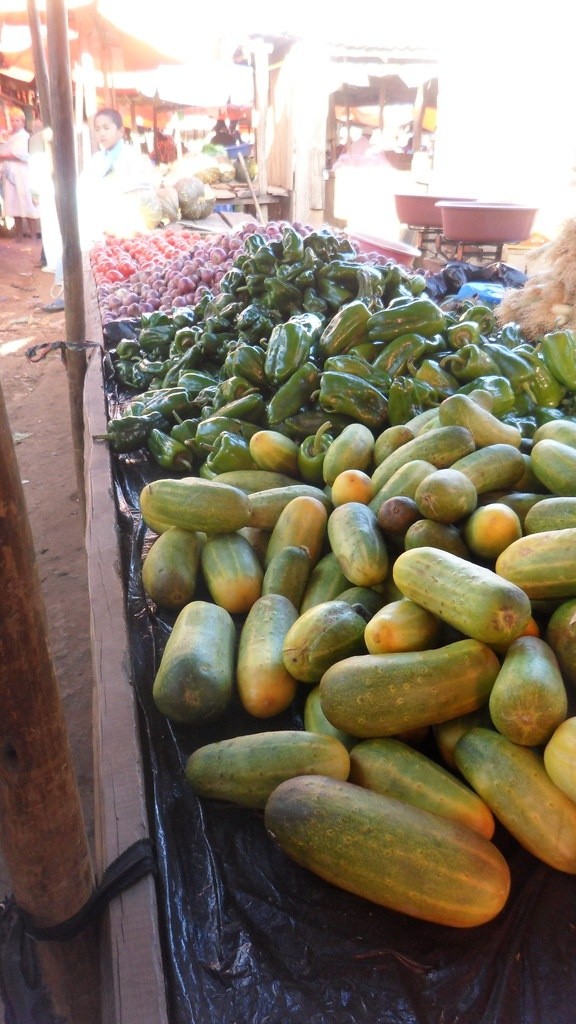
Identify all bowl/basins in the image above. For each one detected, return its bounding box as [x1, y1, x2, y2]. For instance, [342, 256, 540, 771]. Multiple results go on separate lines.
[434, 200, 538, 241]
[349, 229, 422, 268]
[394, 194, 476, 228]
[226, 143, 253, 159]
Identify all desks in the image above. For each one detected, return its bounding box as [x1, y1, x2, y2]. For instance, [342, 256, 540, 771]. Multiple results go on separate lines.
[216, 189, 280, 223]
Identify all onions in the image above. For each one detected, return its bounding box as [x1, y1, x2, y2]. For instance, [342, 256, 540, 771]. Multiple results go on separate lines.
[97, 220, 434, 325]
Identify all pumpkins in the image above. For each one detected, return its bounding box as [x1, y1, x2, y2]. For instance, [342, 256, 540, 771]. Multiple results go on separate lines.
[112, 157, 259, 227]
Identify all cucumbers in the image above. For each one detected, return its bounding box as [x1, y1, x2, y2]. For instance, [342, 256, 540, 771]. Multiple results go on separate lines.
[133, 392, 576, 927]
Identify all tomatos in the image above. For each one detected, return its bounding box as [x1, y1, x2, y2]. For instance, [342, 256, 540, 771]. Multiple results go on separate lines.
[88, 227, 206, 287]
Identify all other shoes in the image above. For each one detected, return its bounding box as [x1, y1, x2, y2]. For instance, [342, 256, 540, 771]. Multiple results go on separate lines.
[42, 299, 64, 313]
[34, 262, 45, 267]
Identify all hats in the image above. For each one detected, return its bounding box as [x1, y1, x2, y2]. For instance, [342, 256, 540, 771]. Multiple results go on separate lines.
[9, 108, 25, 120]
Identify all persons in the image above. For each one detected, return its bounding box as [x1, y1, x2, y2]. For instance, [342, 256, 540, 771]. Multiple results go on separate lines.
[211, 120, 241, 145]
[0, 108, 38, 237]
[42, 108, 150, 312]
[157, 129, 185, 163]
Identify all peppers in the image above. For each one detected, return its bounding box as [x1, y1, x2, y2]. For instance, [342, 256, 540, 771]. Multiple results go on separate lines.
[104, 227, 576, 474]
